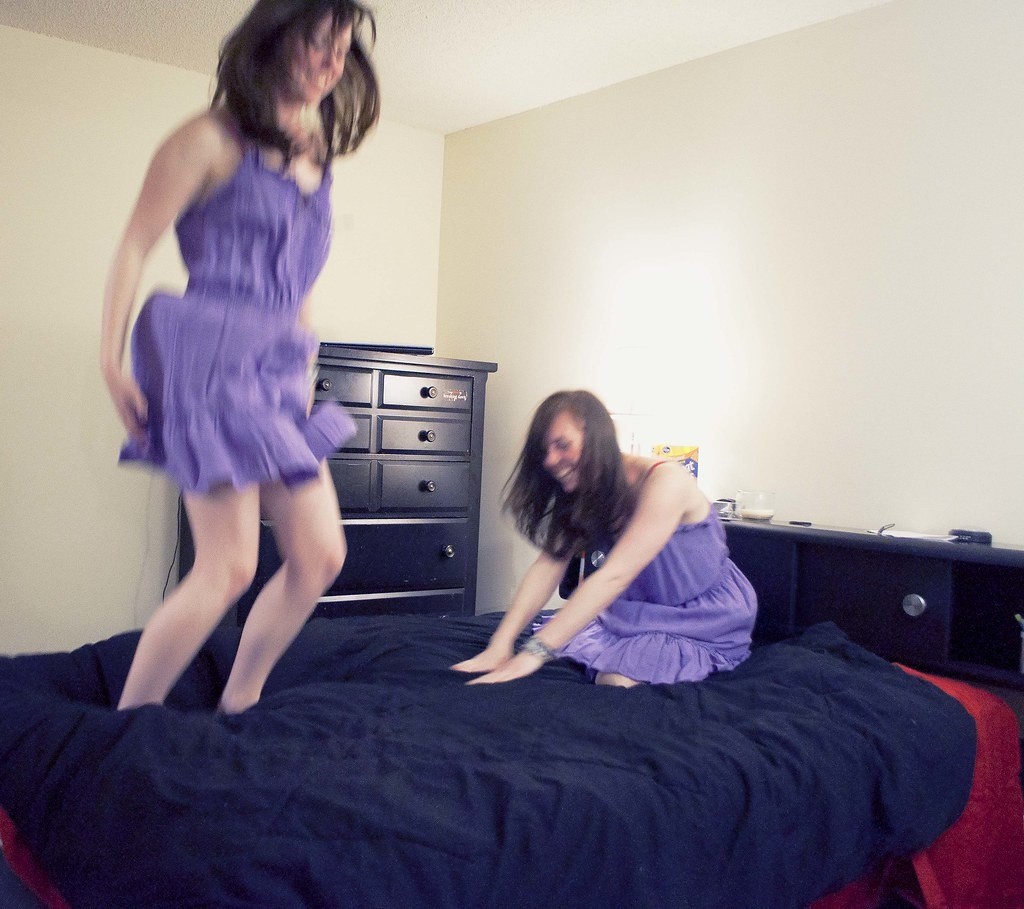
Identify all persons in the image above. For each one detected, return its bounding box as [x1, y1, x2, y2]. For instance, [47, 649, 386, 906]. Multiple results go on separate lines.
[100, 0, 384, 714]
[449, 390, 758, 688]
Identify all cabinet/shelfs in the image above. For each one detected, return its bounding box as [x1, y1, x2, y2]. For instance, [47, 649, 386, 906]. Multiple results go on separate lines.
[179, 346, 499, 615]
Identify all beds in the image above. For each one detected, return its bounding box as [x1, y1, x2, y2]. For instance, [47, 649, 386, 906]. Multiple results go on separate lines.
[0, 523, 1024, 909]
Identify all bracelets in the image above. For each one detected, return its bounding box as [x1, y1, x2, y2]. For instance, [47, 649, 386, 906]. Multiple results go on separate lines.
[520, 636, 557, 662]
